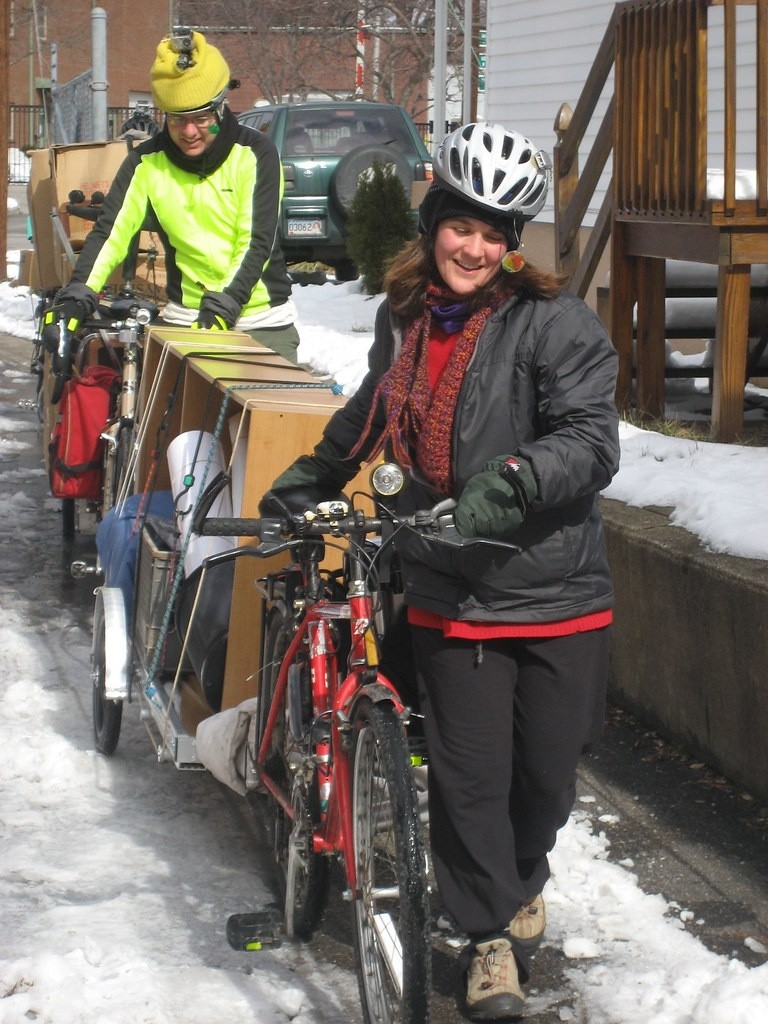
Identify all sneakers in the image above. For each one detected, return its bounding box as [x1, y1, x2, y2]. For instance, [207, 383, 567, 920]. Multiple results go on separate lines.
[508, 892, 546, 947]
[465, 937, 525, 1023]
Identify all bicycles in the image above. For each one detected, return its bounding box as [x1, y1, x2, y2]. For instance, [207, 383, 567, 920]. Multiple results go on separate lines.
[190, 463, 526, 1024]
[78, 294, 194, 520]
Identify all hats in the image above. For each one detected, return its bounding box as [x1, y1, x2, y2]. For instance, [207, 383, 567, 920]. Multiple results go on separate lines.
[149, 32, 231, 115]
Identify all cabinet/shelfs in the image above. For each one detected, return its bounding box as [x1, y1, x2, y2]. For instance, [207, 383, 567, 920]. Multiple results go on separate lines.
[114, 329, 387, 736]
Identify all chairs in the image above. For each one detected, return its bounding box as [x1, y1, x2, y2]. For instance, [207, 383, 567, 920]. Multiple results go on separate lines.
[286, 131, 313, 155]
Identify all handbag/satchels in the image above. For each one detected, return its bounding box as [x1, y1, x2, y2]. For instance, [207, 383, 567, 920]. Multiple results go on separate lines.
[49, 359, 124, 500]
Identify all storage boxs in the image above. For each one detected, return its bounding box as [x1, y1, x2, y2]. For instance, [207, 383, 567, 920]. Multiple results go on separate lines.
[133, 524, 197, 677]
[12, 139, 166, 470]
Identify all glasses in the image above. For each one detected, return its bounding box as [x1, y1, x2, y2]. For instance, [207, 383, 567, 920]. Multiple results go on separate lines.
[166, 106, 218, 126]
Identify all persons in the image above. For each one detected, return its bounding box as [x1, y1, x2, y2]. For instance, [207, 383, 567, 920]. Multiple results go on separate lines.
[122, 113, 162, 138]
[44, 31, 299, 371]
[261, 119, 619, 1024]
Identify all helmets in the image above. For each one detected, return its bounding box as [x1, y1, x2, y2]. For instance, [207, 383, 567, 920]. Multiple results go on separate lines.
[432, 121, 551, 223]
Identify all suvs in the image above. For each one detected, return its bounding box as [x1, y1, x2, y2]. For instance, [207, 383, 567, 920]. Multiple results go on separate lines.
[232, 97, 439, 280]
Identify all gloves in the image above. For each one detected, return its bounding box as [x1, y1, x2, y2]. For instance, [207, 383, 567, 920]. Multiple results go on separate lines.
[191, 290, 242, 332]
[453, 453, 539, 544]
[259, 453, 342, 518]
[39, 282, 99, 353]
